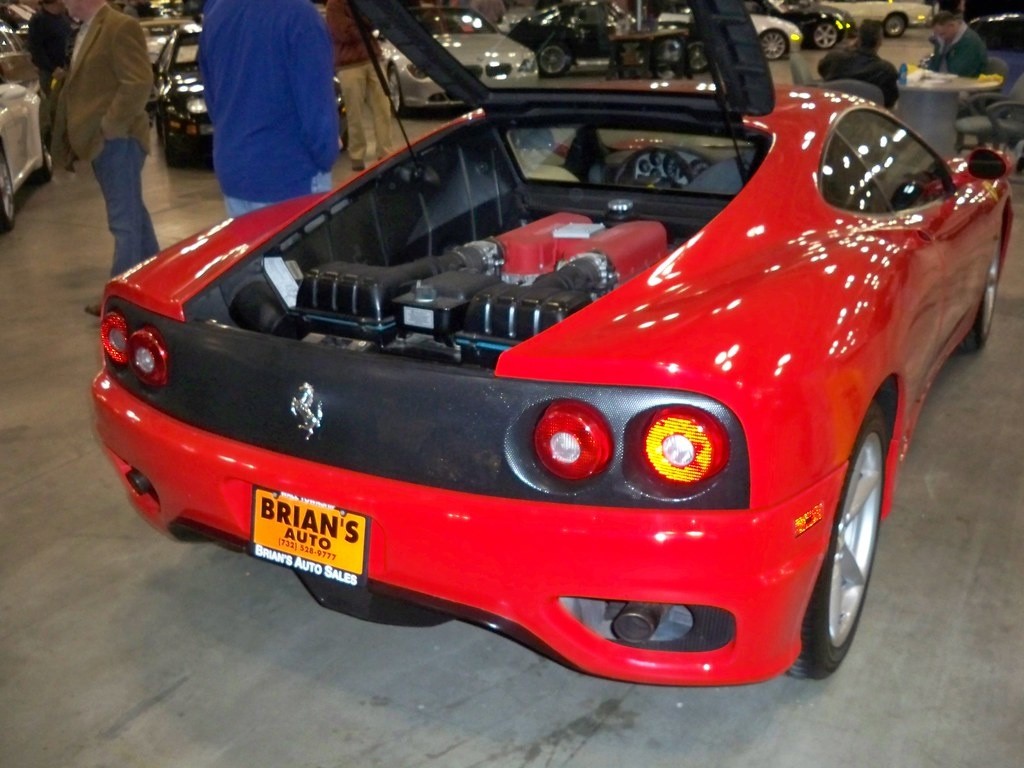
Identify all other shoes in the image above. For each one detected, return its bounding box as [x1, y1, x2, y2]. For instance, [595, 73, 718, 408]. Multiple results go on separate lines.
[351, 159, 366, 171]
[85, 303, 103, 317]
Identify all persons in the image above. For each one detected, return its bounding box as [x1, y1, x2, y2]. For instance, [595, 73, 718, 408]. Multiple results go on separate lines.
[197, 0, 339, 213]
[29, 0, 81, 99]
[123, 0, 140, 19]
[326, 0, 393, 171]
[920, 12, 988, 76]
[512, 128, 578, 182]
[66, 0, 162, 316]
[818, 18, 900, 110]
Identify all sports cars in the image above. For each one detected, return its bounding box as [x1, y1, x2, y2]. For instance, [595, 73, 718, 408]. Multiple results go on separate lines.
[90, 1, 1017, 689]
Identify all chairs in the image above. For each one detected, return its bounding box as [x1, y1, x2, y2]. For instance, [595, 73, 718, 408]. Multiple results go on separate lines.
[429, 18, 464, 34]
[787, 50, 1024, 165]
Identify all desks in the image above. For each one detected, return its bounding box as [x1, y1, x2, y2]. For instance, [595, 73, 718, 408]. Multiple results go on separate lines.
[895, 72, 1001, 162]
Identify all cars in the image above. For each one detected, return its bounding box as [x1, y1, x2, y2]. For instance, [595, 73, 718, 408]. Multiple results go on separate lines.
[0, 0, 941, 234]
